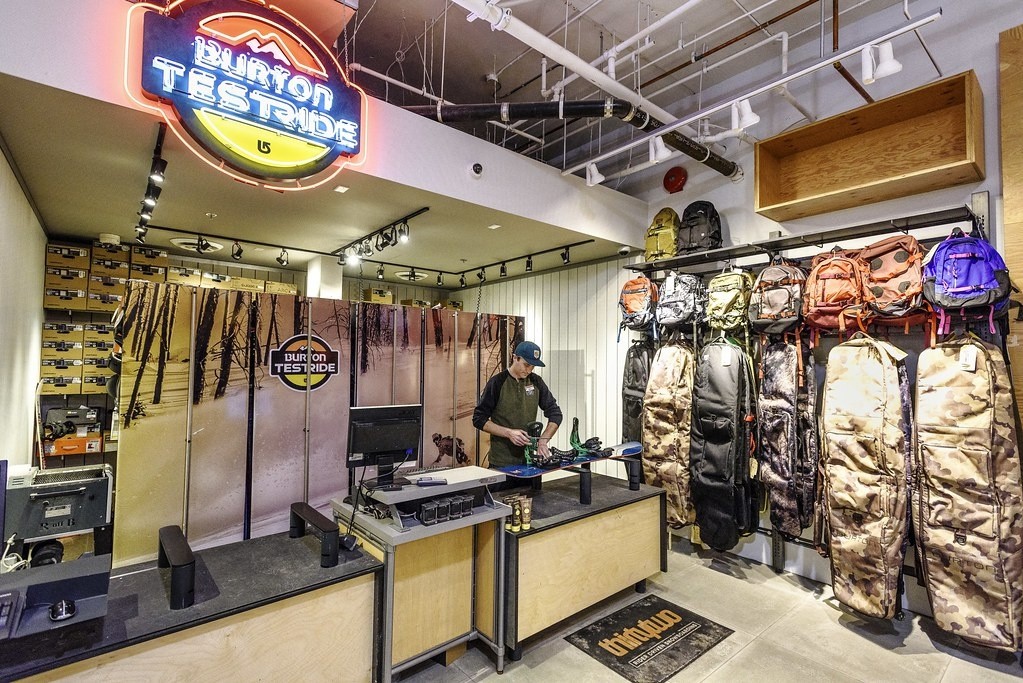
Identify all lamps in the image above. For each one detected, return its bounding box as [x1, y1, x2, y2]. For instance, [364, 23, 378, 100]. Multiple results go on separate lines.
[134, 158, 169, 244]
[738, 99, 760, 129]
[196, 235, 211, 254]
[872, 41, 903, 80]
[231, 241, 243, 260]
[585, 163, 605, 187]
[275, 248, 289, 266]
[336, 221, 573, 289]
[654, 136, 672, 161]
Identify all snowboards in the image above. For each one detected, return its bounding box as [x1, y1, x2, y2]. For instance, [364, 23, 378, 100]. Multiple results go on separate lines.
[495, 440, 644, 478]
[34, 378, 50, 470]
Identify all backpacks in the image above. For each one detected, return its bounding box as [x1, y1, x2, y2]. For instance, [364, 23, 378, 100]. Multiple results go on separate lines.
[922, 227, 1013, 323]
[853, 234, 932, 326]
[706, 264, 754, 331]
[675, 200, 721, 255]
[656, 272, 706, 327]
[620, 277, 658, 331]
[645, 207, 679, 262]
[800, 250, 867, 330]
[746, 255, 809, 335]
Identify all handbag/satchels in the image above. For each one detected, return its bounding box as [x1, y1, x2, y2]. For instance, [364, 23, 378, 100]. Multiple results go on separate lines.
[621, 328, 1023, 652]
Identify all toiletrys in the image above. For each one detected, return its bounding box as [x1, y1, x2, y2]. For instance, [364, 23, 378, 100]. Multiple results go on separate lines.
[501, 493, 533, 533]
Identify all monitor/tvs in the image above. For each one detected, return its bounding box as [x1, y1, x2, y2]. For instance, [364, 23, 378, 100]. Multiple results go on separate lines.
[347, 403, 422, 489]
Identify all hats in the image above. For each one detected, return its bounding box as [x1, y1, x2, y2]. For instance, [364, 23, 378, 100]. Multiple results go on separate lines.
[515, 341, 545, 367]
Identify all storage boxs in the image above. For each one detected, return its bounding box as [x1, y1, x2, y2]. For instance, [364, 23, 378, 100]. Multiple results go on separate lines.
[39, 240, 298, 399]
[351, 288, 462, 312]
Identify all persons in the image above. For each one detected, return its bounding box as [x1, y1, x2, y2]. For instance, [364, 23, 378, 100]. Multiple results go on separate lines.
[472, 340, 563, 494]
